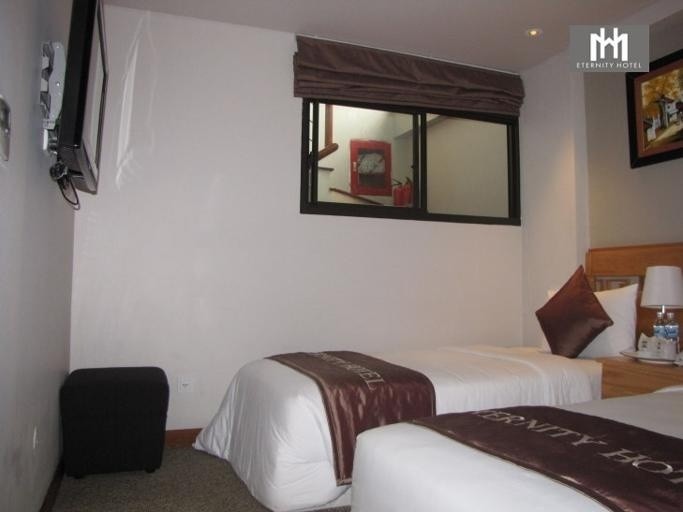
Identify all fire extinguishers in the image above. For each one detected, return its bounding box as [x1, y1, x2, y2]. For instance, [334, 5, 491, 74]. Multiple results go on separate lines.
[389, 178, 412, 207]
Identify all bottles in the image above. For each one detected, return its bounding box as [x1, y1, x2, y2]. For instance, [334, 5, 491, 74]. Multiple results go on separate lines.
[653, 312, 679, 353]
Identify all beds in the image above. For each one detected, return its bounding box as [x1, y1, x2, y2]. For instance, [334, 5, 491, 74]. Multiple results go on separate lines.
[351, 384, 683, 512]
[190, 242, 683, 512]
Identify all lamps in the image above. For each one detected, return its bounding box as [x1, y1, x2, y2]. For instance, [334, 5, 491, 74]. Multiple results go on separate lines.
[639, 265, 683, 314]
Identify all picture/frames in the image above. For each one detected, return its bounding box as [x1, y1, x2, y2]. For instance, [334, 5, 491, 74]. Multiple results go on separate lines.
[625, 48, 683, 169]
[350, 140, 393, 197]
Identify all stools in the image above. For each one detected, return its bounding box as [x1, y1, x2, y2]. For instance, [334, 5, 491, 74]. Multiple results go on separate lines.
[58, 366, 169, 478]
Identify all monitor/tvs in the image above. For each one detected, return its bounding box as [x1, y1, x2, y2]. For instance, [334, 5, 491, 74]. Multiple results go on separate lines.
[59, 0, 110, 195]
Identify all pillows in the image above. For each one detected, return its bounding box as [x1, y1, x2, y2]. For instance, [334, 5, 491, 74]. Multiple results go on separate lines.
[546, 285, 639, 358]
[535, 265, 613, 358]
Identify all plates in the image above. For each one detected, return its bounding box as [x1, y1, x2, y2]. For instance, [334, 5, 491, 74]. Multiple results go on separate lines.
[619, 352, 675, 365]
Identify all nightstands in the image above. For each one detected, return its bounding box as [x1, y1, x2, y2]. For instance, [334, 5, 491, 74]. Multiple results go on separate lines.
[602, 355, 683, 398]
